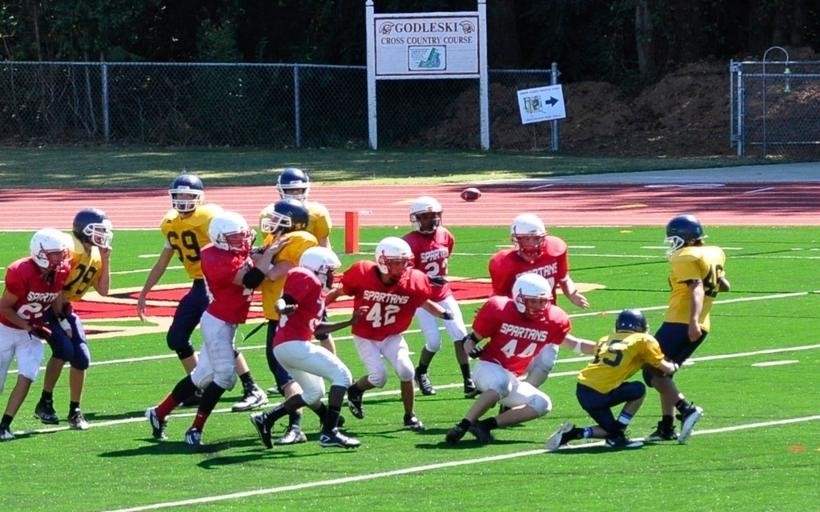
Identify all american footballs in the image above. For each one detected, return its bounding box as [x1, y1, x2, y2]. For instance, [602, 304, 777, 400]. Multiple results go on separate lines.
[461, 188, 481, 201]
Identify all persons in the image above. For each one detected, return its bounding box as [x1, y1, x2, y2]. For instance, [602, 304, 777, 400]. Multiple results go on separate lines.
[326, 236, 455, 431]
[400, 196, 483, 398]
[446, 213, 597, 449]
[0, 228, 72, 441]
[643, 215, 730, 445]
[544, 308, 680, 450]
[34, 208, 114, 432]
[137, 168, 360, 449]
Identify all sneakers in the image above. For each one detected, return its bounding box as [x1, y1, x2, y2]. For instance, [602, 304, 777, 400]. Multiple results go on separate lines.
[185, 427, 203, 445]
[267, 386, 280, 393]
[0, 425, 18, 440]
[446, 423, 466, 445]
[500, 404, 510, 414]
[347, 387, 364, 419]
[468, 420, 496, 444]
[404, 415, 425, 432]
[249, 413, 273, 449]
[274, 426, 308, 444]
[232, 387, 269, 411]
[464, 382, 482, 399]
[338, 416, 345, 427]
[146, 407, 167, 440]
[178, 388, 204, 408]
[32, 401, 58, 425]
[544, 403, 702, 450]
[66, 409, 90, 428]
[319, 427, 361, 449]
[415, 367, 436, 396]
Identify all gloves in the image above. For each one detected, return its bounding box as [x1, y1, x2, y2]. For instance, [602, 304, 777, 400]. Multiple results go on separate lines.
[28, 322, 53, 338]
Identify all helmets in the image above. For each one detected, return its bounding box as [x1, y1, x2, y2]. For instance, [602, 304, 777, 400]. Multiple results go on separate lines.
[510, 214, 547, 263]
[409, 196, 443, 236]
[664, 216, 705, 254]
[169, 174, 204, 217]
[615, 309, 649, 333]
[209, 170, 342, 293]
[30, 228, 73, 276]
[513, 273, 554, 321]
[74, 207, 114, 250]
[375, 237, 416, 280]
